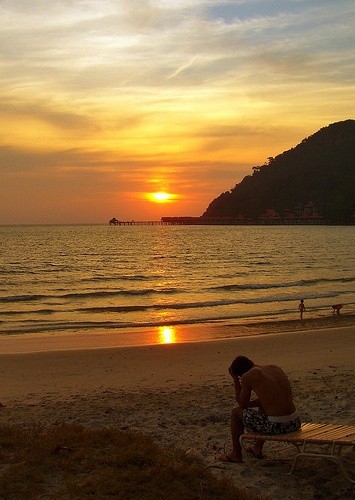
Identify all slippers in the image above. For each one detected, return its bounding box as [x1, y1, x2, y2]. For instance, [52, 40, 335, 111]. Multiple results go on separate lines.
[247, 447, 263, 458]
[219, 454, 243, 463]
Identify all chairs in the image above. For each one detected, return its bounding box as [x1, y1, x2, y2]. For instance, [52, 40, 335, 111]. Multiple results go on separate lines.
[240, 421, 355, 483]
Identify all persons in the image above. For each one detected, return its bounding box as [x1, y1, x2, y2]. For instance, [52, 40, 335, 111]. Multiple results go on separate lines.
[297, 299, 306, 320]
[332, 303, 344, 318]
[216, 354, 303, 463]
